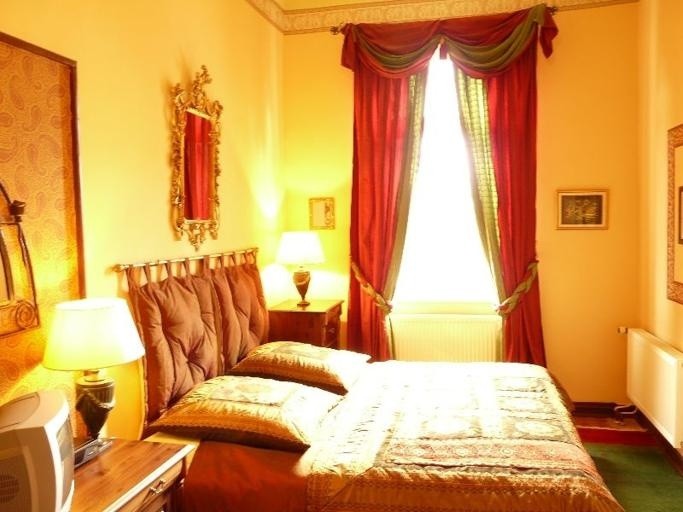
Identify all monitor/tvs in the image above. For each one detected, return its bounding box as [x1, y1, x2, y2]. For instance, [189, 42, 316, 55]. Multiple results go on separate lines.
[0, 387, 75, 512]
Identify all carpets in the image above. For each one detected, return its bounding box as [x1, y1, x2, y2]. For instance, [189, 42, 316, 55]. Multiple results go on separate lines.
[574, 411, 656, 445]
[582, 443, 683, 512]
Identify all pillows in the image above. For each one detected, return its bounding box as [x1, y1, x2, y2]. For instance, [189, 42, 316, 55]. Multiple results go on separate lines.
[149, 375, 345, 454]
[233, 341, 372, 395]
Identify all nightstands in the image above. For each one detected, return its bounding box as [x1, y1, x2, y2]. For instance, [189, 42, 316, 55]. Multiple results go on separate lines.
[69, 438, 195, 512]
[268, 298, 345, 349]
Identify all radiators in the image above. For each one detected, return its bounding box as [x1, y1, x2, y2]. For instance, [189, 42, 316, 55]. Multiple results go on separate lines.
[614, 326, 683, 448]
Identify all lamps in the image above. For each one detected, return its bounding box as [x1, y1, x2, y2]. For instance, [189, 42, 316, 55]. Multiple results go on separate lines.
[268, 231, 325, 306]
[42, 296, 145, 453]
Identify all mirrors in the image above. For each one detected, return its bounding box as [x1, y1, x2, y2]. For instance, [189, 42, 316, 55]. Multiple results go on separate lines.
[170, 65, 224, 249]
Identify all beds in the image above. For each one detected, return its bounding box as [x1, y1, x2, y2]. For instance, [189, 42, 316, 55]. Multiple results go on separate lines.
[117, 248, 625, 512]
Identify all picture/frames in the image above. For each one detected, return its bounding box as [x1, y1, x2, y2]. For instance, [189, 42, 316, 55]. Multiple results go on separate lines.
[309, 197, 335, 230]
[556, 188, 609, 230]
[667, 124, 683, 306]
